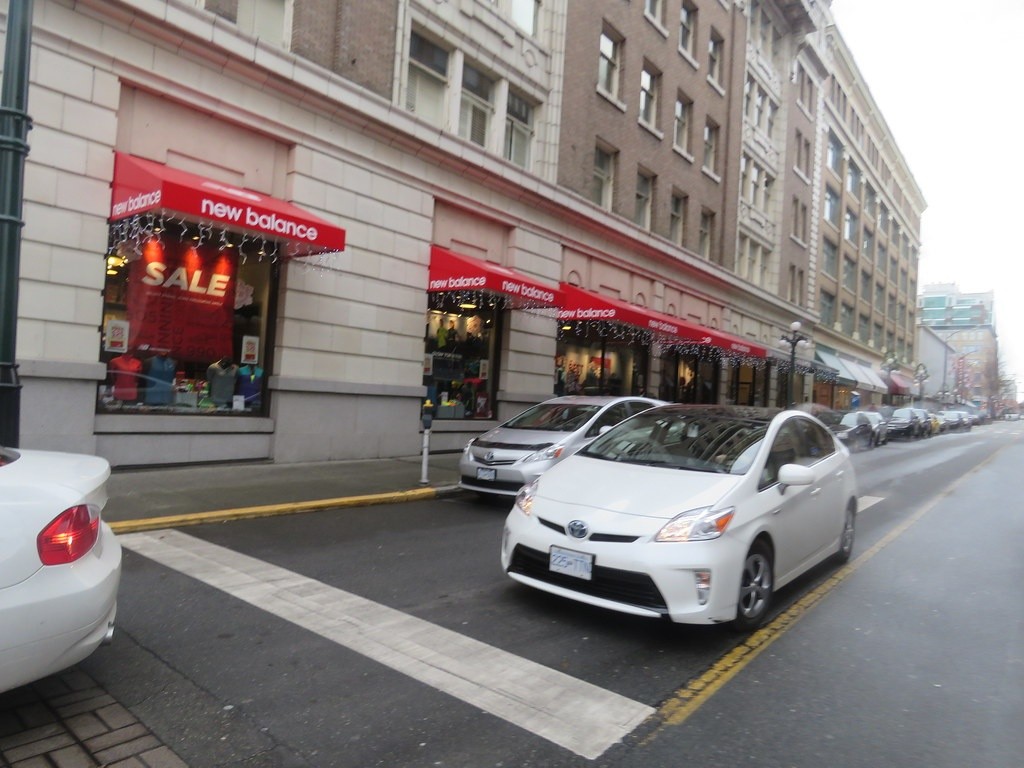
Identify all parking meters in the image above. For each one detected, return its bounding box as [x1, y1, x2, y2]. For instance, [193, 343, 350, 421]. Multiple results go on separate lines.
[420, 400, 437, 483]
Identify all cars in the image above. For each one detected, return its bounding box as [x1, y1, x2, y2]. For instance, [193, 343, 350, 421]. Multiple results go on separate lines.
[460, 394, 689, 498]
[815, 411, 872, 451]
[879, 407, 920, 441]
[99, 371, 260, 411]
[499, 404, 860, 627]
[1, 446, 124, 697]
[913, 408, 993, 436]
[856, 411, 889, 446]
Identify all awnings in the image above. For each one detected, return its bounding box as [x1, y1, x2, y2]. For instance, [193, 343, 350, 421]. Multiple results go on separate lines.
[839, 357, 874, 392]
[558, 281, 766, 358]
[856, 363, 889, 394]
[428, 243, 568, 308]
[109, 152, 346, 258]
[891, 375, 909, 395]
[815, 350, 856, 386]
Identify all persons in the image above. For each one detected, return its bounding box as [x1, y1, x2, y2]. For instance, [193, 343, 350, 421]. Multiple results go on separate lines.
[438, 319, 458, 352]
[110, 347, 143, 405]
[754, 394, 760, 406]
[450, 381, 476, 416]
[835, 400, 840, 410]
[715, 433, 769, 481]
[555, 357, 565, 395]
[680, 377, 687, 400]
[237, 365, 264, 409]
[726, 392, 735, 405]
[566, 361, 616, 394]
[870, 402, 875, 410]
[143, 353, 177, 407]
[207, 355, 239, 408]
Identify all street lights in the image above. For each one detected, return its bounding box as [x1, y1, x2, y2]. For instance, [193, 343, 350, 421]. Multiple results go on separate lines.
[879, 351, 902, 406]
[958, 348, 981, 409]
[779, 322, 812, 408]
[914, 364, 930, 408]
[941, 328, 961, 409]
